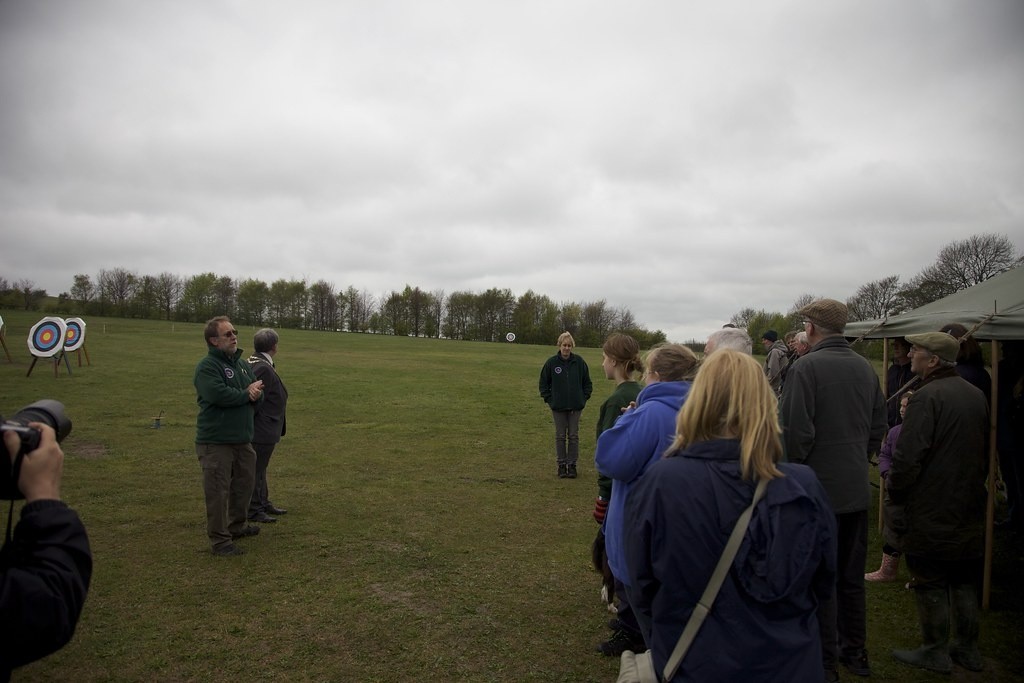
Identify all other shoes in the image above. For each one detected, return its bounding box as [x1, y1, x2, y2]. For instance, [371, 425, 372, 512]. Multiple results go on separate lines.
[840, 648, 872, 676]
[557, 467, 568, 478]
[263, 503, 290, 515]
[211, 542, 246, 556]
[823, 662, 840, 683]
[597, 618, 648, 657]
[568, 466, 577, 478]
[229, 526, 260, 540]
[247, 512, 277, 523]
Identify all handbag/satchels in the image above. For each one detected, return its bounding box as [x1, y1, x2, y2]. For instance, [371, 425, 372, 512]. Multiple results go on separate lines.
[615, 649, 659, 683]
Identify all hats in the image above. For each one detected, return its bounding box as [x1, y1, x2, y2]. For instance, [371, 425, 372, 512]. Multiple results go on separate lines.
[799, 303, 847, 334]
[762, 330, 777, 342]
[905, 332, 960, 363]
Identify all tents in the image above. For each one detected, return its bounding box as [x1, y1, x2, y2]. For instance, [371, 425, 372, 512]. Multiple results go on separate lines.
[842, 261, 1024, 611]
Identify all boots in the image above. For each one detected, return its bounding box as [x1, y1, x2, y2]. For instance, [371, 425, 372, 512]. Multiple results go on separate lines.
[892, 589, 954, 670]
[950, 583, 987, 673]
[863, 553, 901, 582]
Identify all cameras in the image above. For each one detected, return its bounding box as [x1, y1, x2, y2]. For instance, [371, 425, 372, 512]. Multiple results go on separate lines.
[0, 399, 72, 501]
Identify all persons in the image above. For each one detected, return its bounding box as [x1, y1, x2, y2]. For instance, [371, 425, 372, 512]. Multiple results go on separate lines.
[243, 327, 289, 525]
[194, 314, 266, 557]
[538, 331, 593, 479]
[590, 299, 1024, 683]
[884, 332, 991, 672]
[0, 418, 91, 683]
[620, 348, 828, 683]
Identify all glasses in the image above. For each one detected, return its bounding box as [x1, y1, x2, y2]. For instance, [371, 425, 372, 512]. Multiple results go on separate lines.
[802, 321, 811, 324]
[217, 329, 239, 338]
[910, 347, 930, 354]
